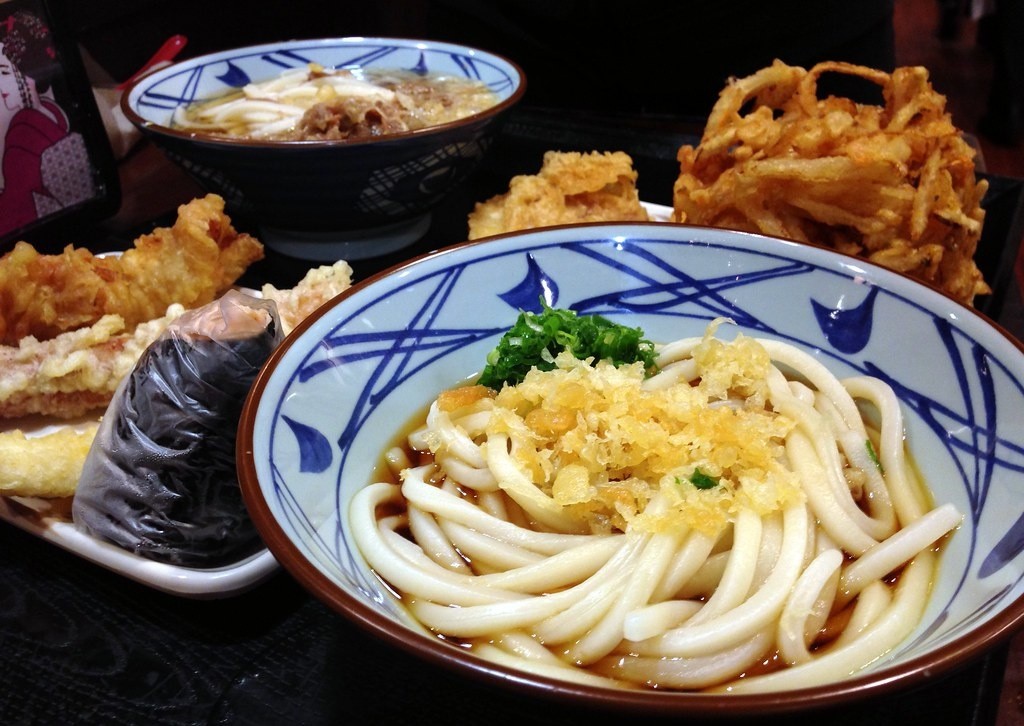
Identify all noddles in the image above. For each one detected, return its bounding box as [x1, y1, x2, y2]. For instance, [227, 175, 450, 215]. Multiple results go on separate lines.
[346, 321, 970, 694]
[170, 66, 411, 146]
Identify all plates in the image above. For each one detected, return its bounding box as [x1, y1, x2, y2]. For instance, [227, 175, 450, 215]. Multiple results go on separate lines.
[1, 250, 280, 596]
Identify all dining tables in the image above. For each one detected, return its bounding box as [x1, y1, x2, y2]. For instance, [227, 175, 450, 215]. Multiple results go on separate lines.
[0, 89, 1022, 725]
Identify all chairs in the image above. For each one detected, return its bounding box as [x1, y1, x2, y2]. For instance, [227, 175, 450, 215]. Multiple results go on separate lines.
[893, 0, 1022, 323]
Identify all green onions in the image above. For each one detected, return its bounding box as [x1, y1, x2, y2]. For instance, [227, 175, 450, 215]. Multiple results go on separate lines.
[479, 295, 657, 392]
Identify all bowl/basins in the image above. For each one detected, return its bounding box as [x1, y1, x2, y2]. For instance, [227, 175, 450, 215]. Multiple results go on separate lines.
[234, 220, 1023, 711]
[120, 38, 526, 224]
